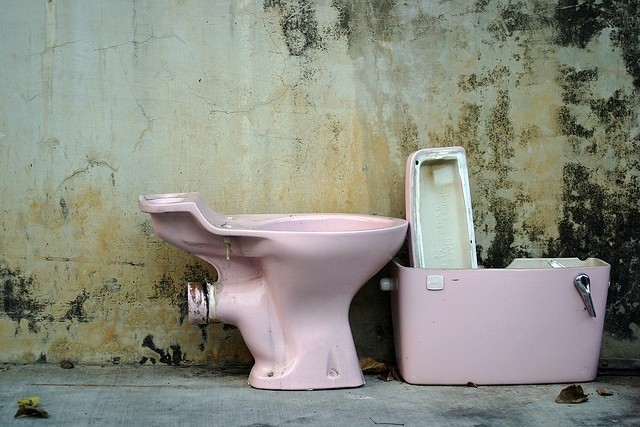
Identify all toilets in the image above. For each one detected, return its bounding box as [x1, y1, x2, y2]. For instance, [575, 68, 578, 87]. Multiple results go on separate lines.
[139, 191, 410, 392]
